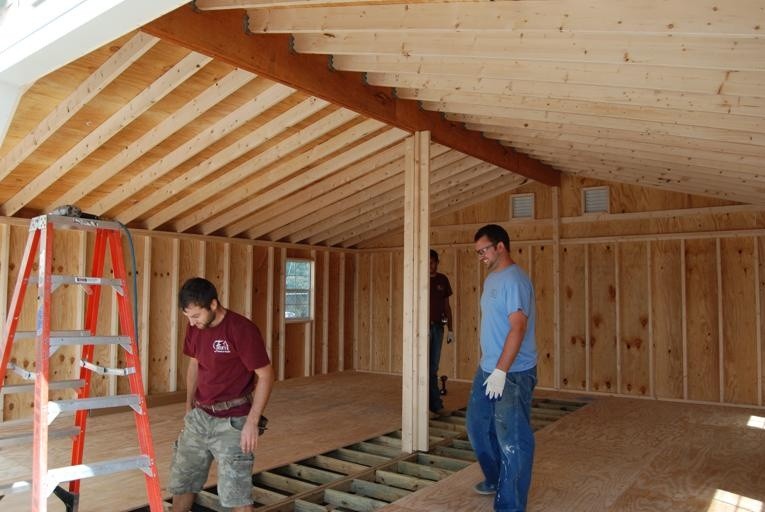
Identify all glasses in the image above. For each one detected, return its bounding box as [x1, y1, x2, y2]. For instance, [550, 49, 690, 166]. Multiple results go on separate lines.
[474, 242, 499, 257]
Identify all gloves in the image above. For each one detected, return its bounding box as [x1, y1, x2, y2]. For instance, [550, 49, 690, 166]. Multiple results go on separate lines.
[447, 331, 455, 345]
[481, 369, 506, 402]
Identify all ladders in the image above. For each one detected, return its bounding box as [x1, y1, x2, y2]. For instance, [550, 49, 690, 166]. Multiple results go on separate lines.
[0, 214, 165, 512]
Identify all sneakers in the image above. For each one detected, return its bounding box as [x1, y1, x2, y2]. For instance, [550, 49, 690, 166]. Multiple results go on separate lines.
[429, 405, 451, 417]
[473, 481, 498, 496]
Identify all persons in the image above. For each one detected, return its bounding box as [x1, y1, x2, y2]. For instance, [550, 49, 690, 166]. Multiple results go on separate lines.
[430, 249, 454, 418]
[465, 224, 537, 512]
[167, 278, 275, 512]
